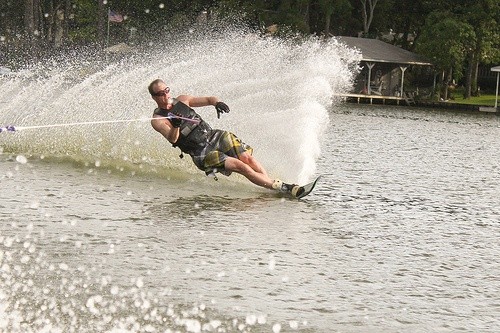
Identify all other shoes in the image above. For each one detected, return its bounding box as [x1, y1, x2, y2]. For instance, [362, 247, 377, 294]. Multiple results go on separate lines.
[272, 179, 305, 198]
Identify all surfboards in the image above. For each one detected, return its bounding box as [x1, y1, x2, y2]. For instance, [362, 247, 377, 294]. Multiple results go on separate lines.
[272, 174, 322, 202]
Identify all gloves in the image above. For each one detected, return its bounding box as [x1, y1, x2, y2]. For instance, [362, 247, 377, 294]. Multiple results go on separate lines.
[215, 100, 230, 119]
[166, 110, 183, 127]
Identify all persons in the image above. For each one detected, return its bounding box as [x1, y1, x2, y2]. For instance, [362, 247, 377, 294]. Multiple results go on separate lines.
[150, 81, 305, 200]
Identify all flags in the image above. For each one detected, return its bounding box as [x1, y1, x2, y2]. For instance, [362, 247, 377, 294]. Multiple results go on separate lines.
[109, 7, 124, 23]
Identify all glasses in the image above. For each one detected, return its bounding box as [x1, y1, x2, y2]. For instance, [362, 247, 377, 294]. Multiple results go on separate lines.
[152, 86, 170, 96]
